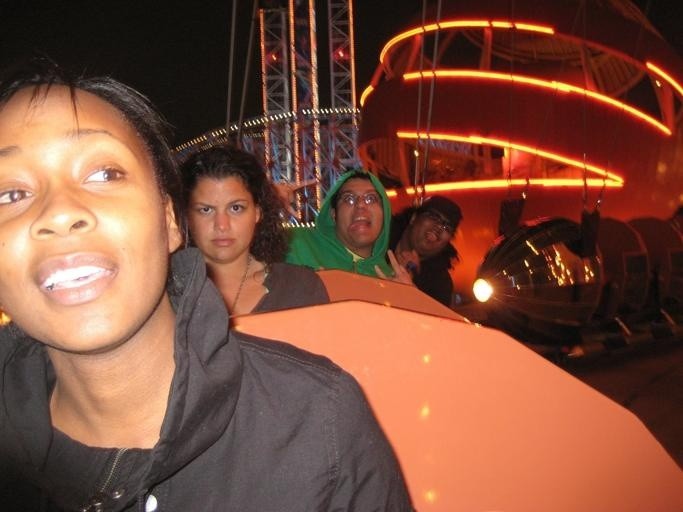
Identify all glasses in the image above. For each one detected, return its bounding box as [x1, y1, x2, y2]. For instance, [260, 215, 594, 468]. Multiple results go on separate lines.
[339, 192, 380, 207]
[423, 211, 456, 232]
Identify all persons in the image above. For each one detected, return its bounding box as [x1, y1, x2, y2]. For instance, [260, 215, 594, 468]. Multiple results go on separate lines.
[0, 49, 415, 512]
[176, 143, 329, 318]
[273, 166, 418, 289]
[386, 193, 464, 308]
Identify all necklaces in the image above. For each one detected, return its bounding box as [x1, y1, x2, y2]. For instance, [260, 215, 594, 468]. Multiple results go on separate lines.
[227, 253, 253, 317]
